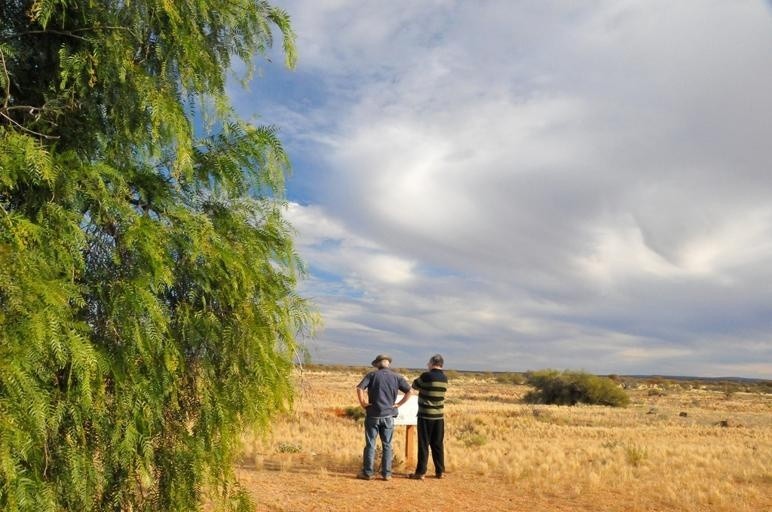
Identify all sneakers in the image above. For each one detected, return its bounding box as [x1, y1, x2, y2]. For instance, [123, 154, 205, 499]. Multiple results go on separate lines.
[436, 474, 444, 478]
[385, 476, 392, 480]
[357, 475, 374, 480]
[409, 474, 425, 479]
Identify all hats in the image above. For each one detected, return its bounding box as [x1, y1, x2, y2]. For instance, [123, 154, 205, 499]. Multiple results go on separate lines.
[371, 355, 392, 367]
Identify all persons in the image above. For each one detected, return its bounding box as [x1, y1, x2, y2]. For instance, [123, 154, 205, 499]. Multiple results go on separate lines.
[357, 355, 413, 481]
[412, 354, 450, 479]
